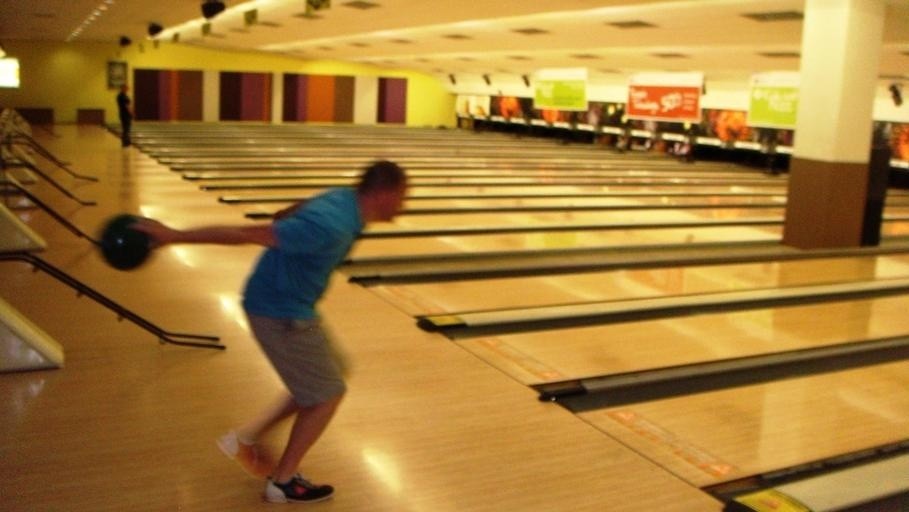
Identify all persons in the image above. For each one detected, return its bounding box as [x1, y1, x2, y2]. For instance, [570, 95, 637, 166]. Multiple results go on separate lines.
[130, 160, 406, 506]
[117, 83, 132, 147]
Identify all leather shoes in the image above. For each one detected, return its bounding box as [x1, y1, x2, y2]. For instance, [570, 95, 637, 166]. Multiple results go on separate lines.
[265, 474, 333, 504]
[215, 429, 275, 482]
